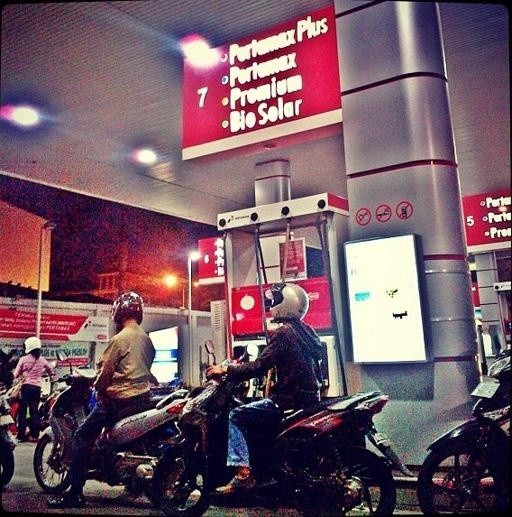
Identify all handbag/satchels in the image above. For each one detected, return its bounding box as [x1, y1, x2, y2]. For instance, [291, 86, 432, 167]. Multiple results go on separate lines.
[4, 374, 26, 400]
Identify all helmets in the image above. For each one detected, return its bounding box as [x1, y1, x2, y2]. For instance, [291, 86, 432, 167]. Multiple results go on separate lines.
[111, 290, 144, 325]
[265, 281, 310, 323]
[23, 336, 42, 356]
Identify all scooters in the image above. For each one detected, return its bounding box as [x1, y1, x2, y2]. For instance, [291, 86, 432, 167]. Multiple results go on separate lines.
[4, 360, 62, 439]
[0, 397, 18, 489]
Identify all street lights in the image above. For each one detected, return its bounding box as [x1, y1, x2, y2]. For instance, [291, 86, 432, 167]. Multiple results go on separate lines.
[182, 250, 200, 387]
[35, 221, 57, 339]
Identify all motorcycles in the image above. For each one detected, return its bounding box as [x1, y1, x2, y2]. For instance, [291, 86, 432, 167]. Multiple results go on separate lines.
[142, 339, 415, 517]
[417, 349, 512, 517]
[32, 348, 194, 494]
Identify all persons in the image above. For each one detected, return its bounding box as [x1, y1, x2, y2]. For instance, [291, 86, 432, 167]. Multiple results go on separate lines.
[206, 282, 322, 494]
[12, 335, 57, 442]
[45, 290, 156, 508]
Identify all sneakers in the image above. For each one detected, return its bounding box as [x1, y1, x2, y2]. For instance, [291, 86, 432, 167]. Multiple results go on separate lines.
[46, 488, 87, 509]
[13, 434, 38, 443]
[214, 471, 256, 495]
